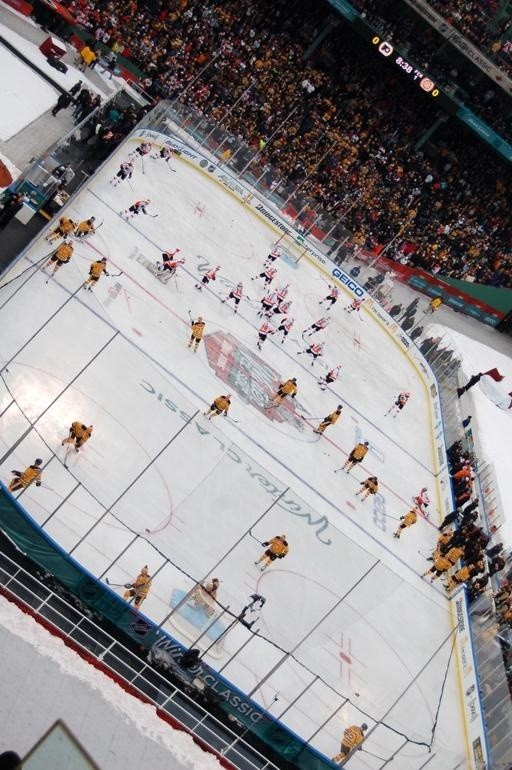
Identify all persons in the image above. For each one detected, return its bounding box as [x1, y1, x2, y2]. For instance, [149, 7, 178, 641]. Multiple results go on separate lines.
[332, 723, 369, 762]
[0, 0, 512, 620]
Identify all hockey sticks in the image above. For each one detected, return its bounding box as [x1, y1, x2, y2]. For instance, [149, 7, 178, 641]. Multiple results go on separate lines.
[359, 747, 394, 762]
[246, 295, 262, 303]
[69, 233, 86, 244]
[174, 274, 184, 294]
[164, 155, 176, 172]
[302, 332, 312, 346]
[188, 310, 194, 323]
[381, 511, 403, 522]
[301, 415, 328, 422]
[46, 262, 64, 284]
[106, 578, 135, 587]
[89, 221, 103, 233]
[137, 209, 159, 217]
[125, 175, 134, 192]
[63, 431, 72, 469]
[89, 271, 122, 276]
[249, 528, 269, 549]
[440, 574, 455, 580]
[213, 407, 238, 423]
[334, 460, 359, 473]
[141, 154, 146, 176]
[418, 550, 436, 562]
[356, 308, 366, 322]
